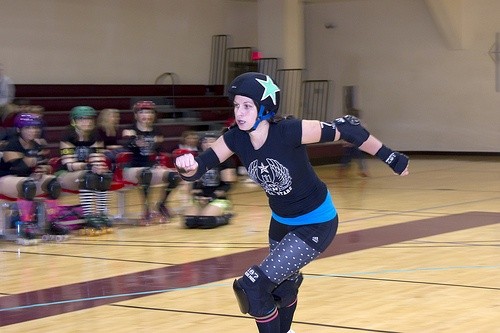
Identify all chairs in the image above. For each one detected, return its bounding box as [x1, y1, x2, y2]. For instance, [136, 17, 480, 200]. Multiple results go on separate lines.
[0, 149, 197, 241]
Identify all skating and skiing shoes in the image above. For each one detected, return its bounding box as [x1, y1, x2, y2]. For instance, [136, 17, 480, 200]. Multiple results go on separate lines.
[42, 222, 70, 243]
[79, 215, 114, 237]
[131, 211, 166, 226]
[16, 228, 39, 246]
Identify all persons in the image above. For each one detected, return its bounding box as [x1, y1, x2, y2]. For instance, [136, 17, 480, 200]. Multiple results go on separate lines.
[171, 73, 410, 333]
[0, 72, 368, 240]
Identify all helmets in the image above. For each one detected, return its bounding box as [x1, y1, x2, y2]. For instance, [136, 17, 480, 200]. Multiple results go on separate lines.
[14, 113, 41, 126]
[228, 72, 280, 112]
[68, 106, 98, 120]
[133, 100, 157, 111]
[197, 131, 218, 149]
